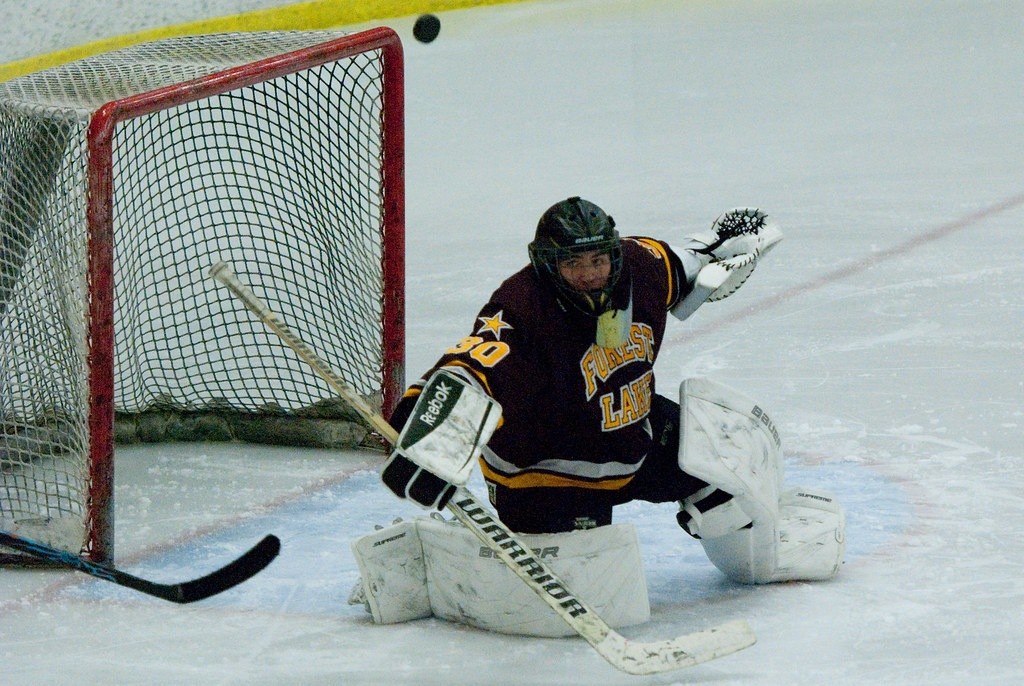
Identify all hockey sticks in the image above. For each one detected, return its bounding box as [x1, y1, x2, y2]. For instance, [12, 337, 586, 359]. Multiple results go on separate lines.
[0, 530, 283, 608]
[209, 262, 760, 677]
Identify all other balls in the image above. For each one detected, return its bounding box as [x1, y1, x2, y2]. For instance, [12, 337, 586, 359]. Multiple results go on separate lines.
[412, 13, 441, 43]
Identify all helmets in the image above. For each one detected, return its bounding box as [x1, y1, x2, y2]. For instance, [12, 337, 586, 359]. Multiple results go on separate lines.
[526, 196, 624, 319]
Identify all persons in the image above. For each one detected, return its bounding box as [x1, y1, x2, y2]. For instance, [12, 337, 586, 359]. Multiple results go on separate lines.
[351, 196, 843, 638]
